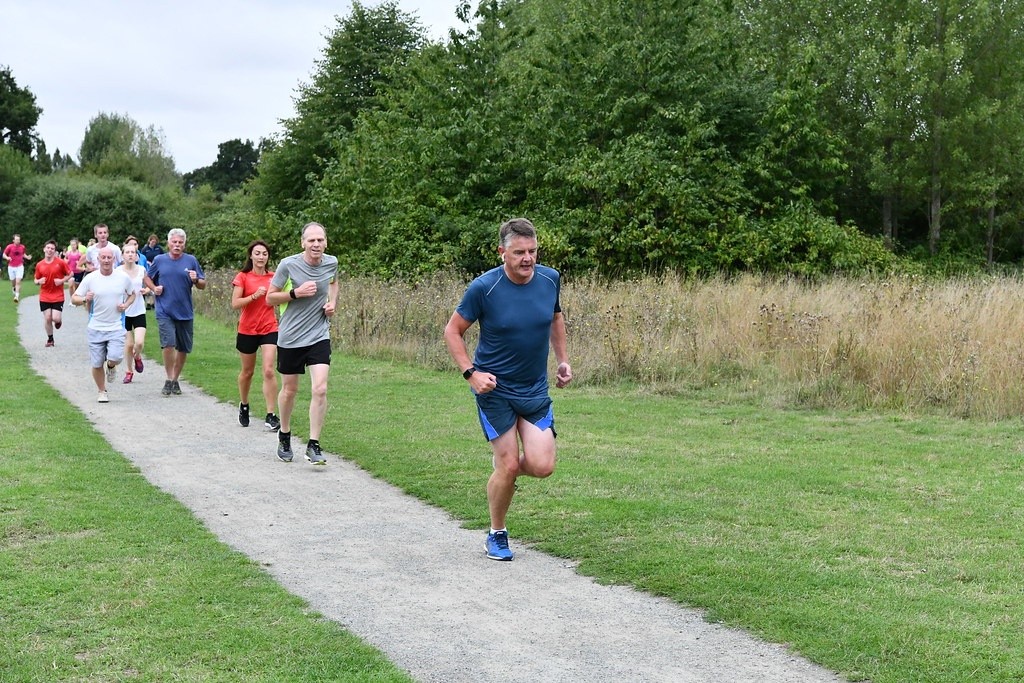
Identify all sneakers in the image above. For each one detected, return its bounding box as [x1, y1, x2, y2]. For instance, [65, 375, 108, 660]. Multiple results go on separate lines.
[134, 352, 143, 373]
[238, 402, 250, 427]
[484, 531, 513, 561]
[171, 381, 182, 395]
[98, 390, 109, 402]
[123, 371, 133, 383]
[305, 443, 327, 465]
[276, 428, 293, 462]
[162, 380, 172, 395]
[106, 360, 116, 383]
[265, 413, 281, 430]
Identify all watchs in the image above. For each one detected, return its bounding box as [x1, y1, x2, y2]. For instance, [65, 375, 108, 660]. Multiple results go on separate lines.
[462, 368, 476, 380]
[290, 289, 297, 300]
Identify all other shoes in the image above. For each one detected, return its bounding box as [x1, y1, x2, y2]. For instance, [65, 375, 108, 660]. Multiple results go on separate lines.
[14, 297, 18, 303]
[55, 321, 61, 329]
[12, 286, 16, 292]
[146, 304, 151, 310]
[45, 340, 54, 348]
[69, 303, 76, 308]
[151, 304, 155, 309]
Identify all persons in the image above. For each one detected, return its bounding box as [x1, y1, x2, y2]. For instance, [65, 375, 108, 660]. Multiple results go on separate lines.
[71, 248, 136, 403]
[231, 240, 281, 430]
[145, 228, 206, 395]
[445, 218, 572, 560]
[59, 223, 148, 311]
[265, 222, 338, 465]
[117, 244, 150, 383]
[141, 235, 164, 310]
[2, 234, 32, 303]
[34, 240, 74, 347]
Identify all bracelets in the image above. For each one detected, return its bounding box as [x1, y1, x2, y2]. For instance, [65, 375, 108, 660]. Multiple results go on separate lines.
[194, 279, 198, 284]
[251, 294, 257, 301]
[83, 296, 86, 303]
